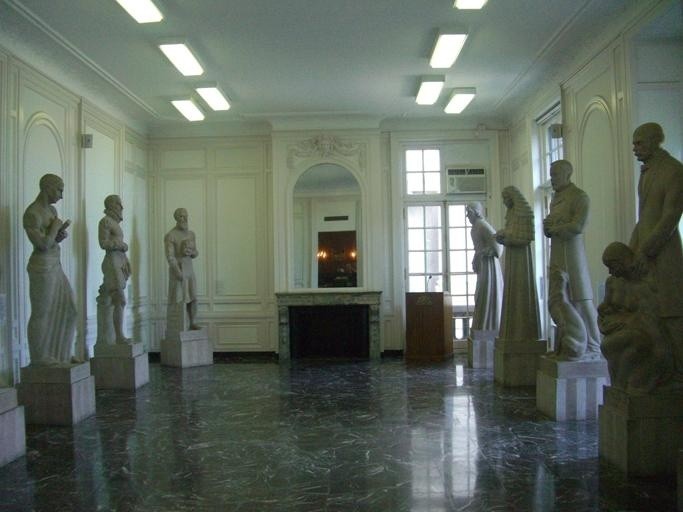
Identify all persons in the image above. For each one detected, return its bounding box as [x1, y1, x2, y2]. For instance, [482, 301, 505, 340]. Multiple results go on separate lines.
[96, 191, 139, 349]
[162, 206, 204, 334]
[622, 121, 683, 378]
[590, 238, 675, 393]
[23, 172, 81, 369]
[464, 200, 506, 335]
[495, 184, 544, 342]
[596, 302, 633, 335]
[539, 157, 599, 359]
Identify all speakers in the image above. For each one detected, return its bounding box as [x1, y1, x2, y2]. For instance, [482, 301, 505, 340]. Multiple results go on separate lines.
[81, 133, 93, 148]
[551, 124, 562, 138]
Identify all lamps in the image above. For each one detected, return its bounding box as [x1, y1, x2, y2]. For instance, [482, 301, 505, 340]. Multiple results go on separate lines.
[415, 0, 488, 115]
[115, 0, 232, 122]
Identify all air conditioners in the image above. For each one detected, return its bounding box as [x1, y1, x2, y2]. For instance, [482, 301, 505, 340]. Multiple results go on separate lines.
[446, 166, 487, 195]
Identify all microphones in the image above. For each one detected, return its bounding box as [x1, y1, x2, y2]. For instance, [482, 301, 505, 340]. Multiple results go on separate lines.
[426, 275, 432, 292]
